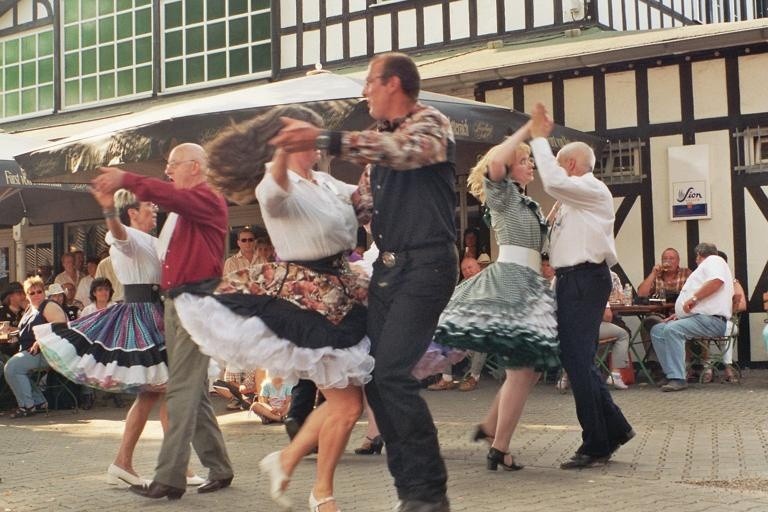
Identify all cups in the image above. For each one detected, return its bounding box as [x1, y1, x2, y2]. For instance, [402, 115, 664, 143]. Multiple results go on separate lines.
[660, 263, 673, 271]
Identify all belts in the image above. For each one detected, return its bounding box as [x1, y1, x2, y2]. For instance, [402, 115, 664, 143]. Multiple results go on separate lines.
[712, 315, 727, 322]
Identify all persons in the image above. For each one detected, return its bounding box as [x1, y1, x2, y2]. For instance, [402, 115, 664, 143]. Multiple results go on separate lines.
[87, 178, 206, 491]
[529, 101, 636, 470]
[253, 108, 372, 512]
[411, 229, 746, 390]
[90, 141, 235, 500]
[266, 52, 461, 512]
[466, 107, 561, 471]
[1, 228, 385, 454]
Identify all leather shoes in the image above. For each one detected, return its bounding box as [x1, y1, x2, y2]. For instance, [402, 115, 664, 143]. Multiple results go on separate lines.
[108, 463, 234, 500]
[428, 376, 478, 390]
[560, 428, 635, 468]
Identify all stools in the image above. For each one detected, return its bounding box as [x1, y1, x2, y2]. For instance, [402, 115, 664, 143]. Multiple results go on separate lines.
[592, 337, 619, 386]
[29, 367, 78, 415]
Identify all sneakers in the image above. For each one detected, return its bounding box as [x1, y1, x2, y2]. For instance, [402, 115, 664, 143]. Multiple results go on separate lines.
[227, 400, 241, 409]
[656, 378, 687, 392]
[607, 372, 628, 389]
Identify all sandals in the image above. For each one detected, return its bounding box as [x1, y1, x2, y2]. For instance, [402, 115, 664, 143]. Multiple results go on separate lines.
[10, 402, 49, 418]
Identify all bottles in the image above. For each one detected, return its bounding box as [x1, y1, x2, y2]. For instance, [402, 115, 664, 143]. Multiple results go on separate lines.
[624, 284, 631, 306]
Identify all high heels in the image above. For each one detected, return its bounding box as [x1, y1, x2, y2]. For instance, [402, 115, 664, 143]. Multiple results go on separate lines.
[310, 488, 340, 512]
[259, 451, 290, 508]
[475, 425, 524, 470]
[355, 435, 383, 454]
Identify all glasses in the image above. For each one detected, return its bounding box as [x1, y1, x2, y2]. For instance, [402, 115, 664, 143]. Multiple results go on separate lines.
[30, 290, 42, 295]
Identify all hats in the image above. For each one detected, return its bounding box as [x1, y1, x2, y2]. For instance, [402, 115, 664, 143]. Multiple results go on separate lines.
[0, 282, 24, 300]
[45, 283, 65, 297]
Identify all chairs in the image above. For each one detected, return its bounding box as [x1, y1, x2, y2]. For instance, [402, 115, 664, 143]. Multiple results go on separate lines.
[679, 293, 751, 383]
